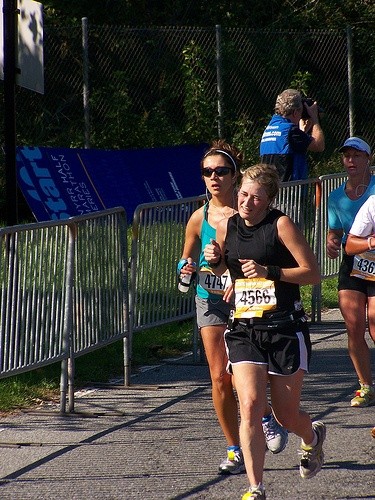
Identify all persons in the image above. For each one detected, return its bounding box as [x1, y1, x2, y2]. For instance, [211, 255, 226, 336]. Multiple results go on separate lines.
[177, 140, 288, 474]
[260, 89, 324, 232]
[326, 136, 375, 407]
[345, 195, 375, 256]
[202, 163, 326, 500]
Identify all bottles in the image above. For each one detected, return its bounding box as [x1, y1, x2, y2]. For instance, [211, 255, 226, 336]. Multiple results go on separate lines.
[178, 262, 196, 293]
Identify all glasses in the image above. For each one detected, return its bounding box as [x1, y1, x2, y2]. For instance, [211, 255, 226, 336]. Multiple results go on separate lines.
[201, 167, 232, 177]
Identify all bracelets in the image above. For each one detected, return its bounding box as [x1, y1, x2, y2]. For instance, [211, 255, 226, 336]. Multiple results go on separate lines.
[265, 265, 281, 282]
[368, 237, 375, 251]
[207, 254, 223, 268]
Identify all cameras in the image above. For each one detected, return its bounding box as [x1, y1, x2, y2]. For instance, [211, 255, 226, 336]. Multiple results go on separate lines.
[301, 97, 314, 120]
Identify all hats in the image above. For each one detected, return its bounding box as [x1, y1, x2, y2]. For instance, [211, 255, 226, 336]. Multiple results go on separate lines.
[338, 137, 371, 155]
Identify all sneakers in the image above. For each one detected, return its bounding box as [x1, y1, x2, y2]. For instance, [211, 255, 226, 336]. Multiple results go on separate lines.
[300, 420, 326, 479]
[218, 446, 246, 475]
[242, 482, 265, 500]
[350, 381, 375, 407]
[261, 413, 288, 454]
